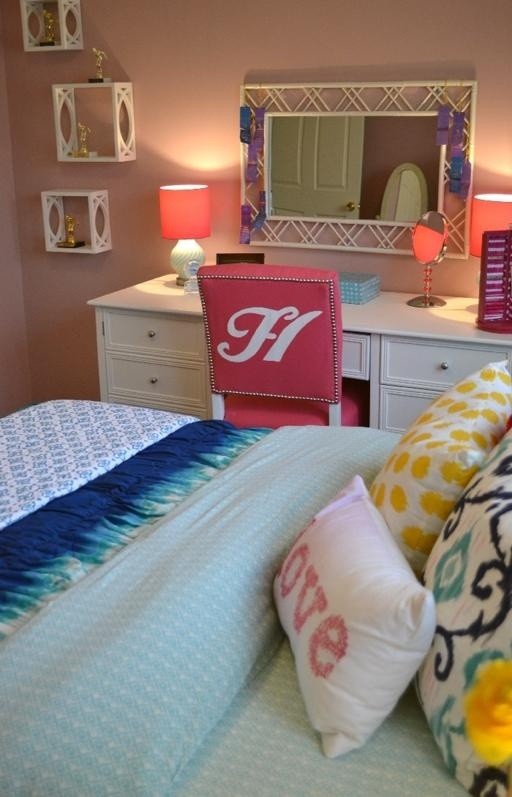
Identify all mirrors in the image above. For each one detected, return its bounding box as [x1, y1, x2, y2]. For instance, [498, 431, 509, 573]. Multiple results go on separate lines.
[235, 78, 479, 263]
[406, 211, 452, 311]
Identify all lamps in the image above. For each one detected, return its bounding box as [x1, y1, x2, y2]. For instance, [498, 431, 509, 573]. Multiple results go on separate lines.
[464, 194, 512, 268]
[159, 183, 215, 289]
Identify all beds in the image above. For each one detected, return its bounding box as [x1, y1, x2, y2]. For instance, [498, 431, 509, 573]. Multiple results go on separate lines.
[0, 398, 511, 797]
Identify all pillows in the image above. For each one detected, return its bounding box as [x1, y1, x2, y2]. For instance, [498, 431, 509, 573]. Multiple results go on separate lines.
[272, 475, 436, 762]
[366, 359, 512, 585]
[410, 427, 512, 796]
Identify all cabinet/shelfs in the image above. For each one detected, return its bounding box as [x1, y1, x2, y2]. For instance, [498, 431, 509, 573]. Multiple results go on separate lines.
[83, 270, 512, 439]
